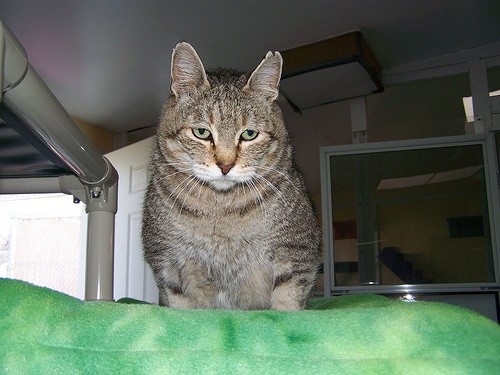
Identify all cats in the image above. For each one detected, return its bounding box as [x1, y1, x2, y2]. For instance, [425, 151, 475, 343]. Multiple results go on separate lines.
[141, 39, 323, 309]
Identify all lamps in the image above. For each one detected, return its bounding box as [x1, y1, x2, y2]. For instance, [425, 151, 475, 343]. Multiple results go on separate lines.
[278, 29, 385, 117]
